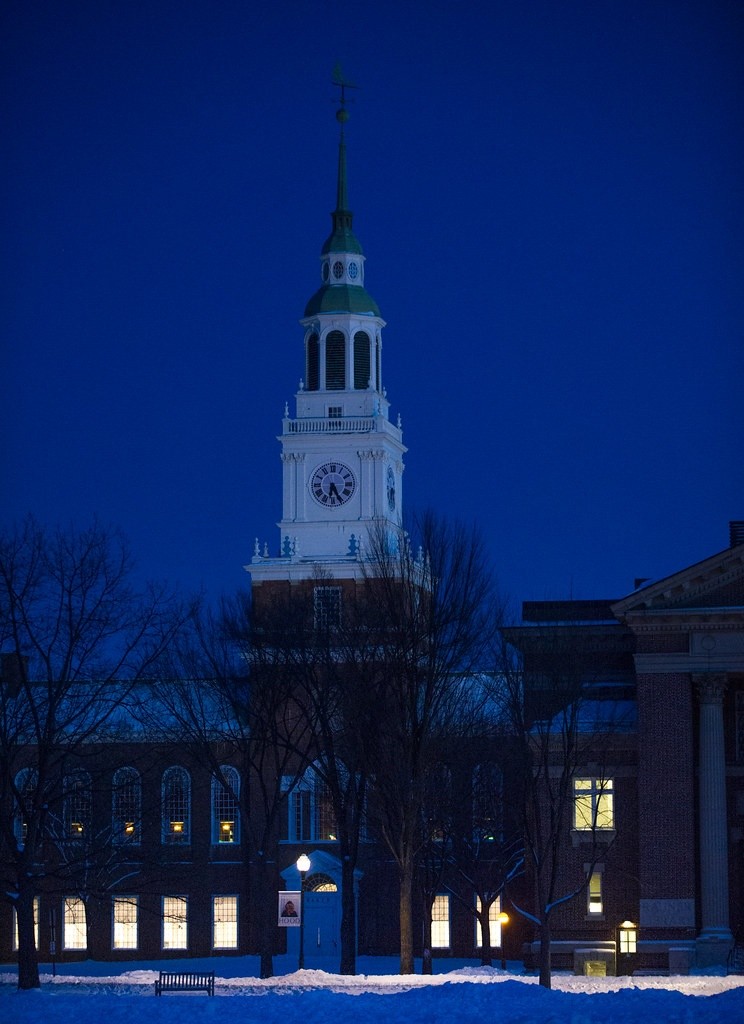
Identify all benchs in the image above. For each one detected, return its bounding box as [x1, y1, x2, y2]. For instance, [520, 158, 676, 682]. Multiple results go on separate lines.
[154, 970, 215, 996]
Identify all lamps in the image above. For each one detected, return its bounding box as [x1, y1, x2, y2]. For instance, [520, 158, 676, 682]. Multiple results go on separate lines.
[618, 910, 636, 959]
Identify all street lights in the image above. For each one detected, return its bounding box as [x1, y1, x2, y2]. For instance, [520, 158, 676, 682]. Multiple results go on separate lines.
[296, 853, 312, 970]
[499, 913, 510, 971]
